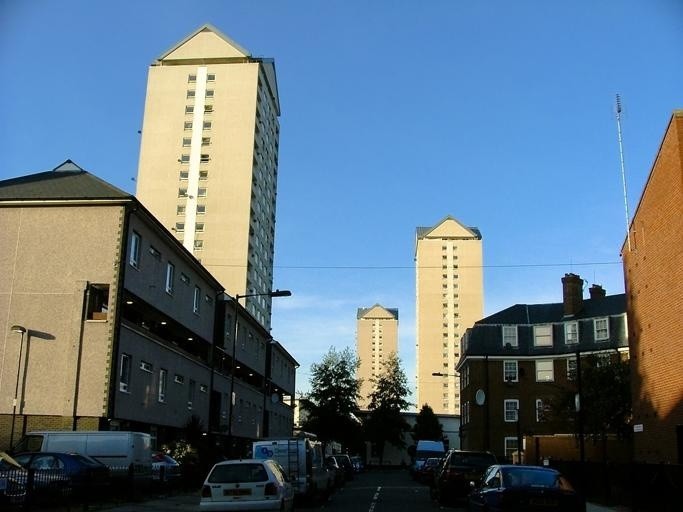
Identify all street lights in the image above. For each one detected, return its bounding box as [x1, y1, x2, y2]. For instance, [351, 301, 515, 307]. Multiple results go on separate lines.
[432, 373, 490, 451]
[10, 326, 26, 450]
[227, 290, 291, 460]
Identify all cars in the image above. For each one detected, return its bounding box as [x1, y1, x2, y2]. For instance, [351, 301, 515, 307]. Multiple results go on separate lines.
[199, 437, 367, 512]
[0, 430, 182, 512]
[412, 440, 578, 512]
